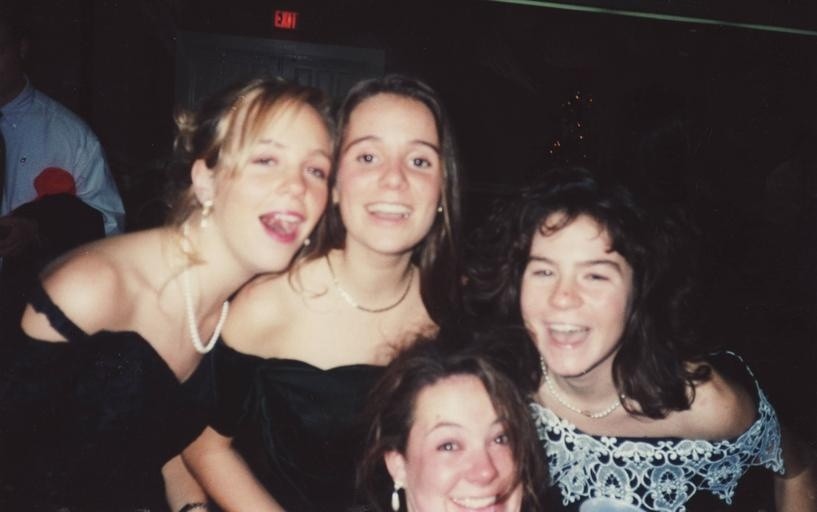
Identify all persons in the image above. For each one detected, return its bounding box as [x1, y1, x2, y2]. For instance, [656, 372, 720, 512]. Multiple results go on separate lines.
[461, 173, 817, 512]
[354, 331, 551, 512]
[179, 72, 478, 512]
[0, 9, 127, 325]
[0, 75, 339, 512]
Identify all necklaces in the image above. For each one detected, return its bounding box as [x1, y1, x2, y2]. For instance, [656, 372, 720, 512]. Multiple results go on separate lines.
[326, 253, 414, 313]
[182, 219, 230, 354]
[540, 357, 627, 419]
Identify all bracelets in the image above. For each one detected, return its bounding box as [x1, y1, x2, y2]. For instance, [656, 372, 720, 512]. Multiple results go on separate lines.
[179, 502, 212, 512]
